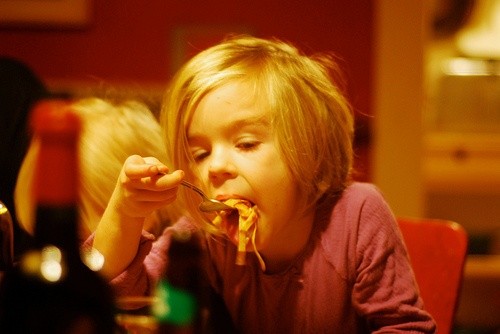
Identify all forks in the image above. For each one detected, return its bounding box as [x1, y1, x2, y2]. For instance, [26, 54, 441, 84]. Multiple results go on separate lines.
[157, 172, 236, 212]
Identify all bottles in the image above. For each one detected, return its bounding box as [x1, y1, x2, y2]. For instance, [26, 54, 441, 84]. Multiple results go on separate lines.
[1, 109, 114, 334]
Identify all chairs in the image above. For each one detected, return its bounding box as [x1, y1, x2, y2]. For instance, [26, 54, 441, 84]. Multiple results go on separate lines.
[393, 215, 470, 334]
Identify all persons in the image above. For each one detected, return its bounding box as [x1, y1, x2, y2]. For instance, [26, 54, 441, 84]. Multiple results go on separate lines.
[0, 54, 181, 247]
[79, 36, 436, 334]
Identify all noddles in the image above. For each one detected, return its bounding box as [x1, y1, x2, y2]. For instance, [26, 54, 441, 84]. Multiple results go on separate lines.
[211, 199, 266, 272]
